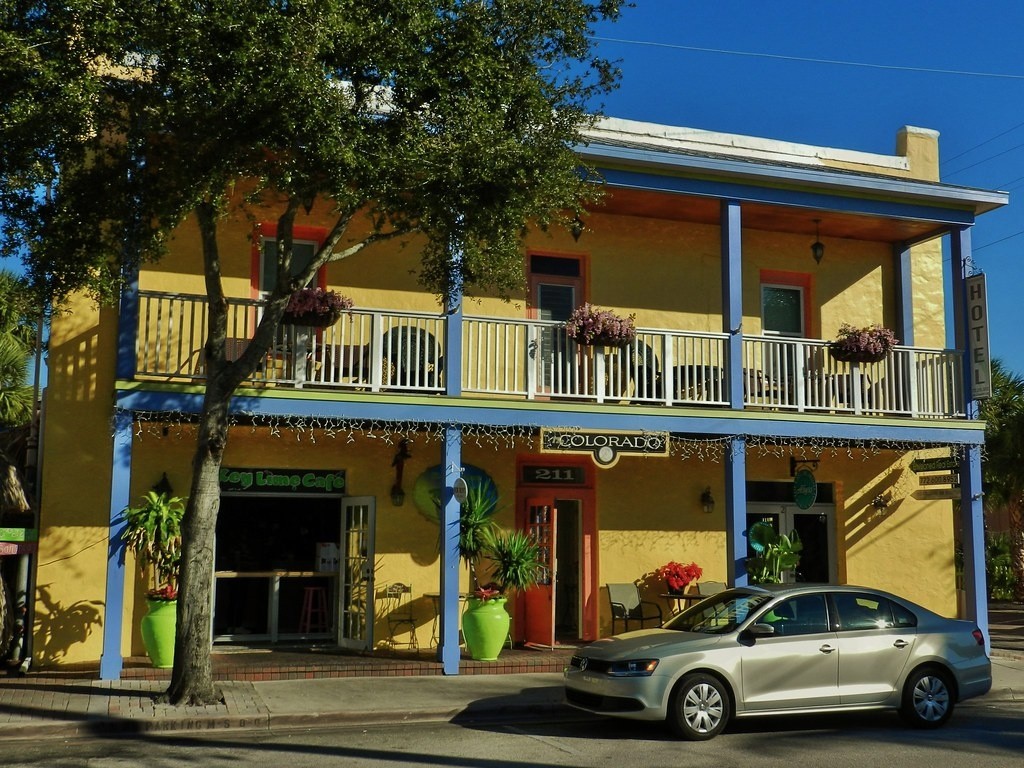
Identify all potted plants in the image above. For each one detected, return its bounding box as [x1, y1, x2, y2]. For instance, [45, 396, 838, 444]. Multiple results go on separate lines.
[121, 488, 189, 668]
[430, 474, 550, 662]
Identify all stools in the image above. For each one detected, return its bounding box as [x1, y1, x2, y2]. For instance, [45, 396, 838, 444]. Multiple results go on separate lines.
[299, 586, 331, 644]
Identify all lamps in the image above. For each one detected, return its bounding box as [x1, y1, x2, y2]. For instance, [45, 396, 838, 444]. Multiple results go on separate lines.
[811, 218, 826, 266]
[700, 486, 716, 514]
[389, 482, 406, 507]
[572, 216, 585, 244]
[153, 471, 174, 504]
[873, 493, 888, 517]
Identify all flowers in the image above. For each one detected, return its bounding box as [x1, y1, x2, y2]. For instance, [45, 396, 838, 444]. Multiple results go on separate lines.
[826, 322, 900, 357]
[564, 301, 638, 345]
[283, 287, 355, 324]
[655, 560, 703, 590]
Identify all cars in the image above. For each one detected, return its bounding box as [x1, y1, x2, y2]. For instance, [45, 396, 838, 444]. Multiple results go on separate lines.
[561, 584, 994, 741]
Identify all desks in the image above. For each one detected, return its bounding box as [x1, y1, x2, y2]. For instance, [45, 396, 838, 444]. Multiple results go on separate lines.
[268, 343, 331, 388]
[423, 591, 469, 651]
[657, 594, 712, 617]
[214, 571, 340, 646]
[672, 365, 725, 408]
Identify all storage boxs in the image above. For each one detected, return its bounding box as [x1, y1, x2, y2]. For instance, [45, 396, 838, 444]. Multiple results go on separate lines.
[315, 543, 341, 573]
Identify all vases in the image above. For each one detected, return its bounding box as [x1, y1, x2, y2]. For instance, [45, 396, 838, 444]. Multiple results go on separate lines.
[279, 310, 342, 329]
[665, 580, 685, 595]
[573, 325, 629, 348]
[829, 345, 887, 363]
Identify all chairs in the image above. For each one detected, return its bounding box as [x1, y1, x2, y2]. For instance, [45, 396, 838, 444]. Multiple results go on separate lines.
[772, 602, 795, 635]
[605, 582, 663, 637]
[381, 326, 443, 395]
[877, 603, 899, 624]
[326, 344, 369, 391]
[696, 580, 729, 627]
[621, 339, 662, 406]
[386, 583, 420, 653]
[225, 337, 263, 385]
[795, 596, 826, 634]
[743, 368, 792, 410]
[832, 374, 872, 415]
[505, 617, 513, 651]
